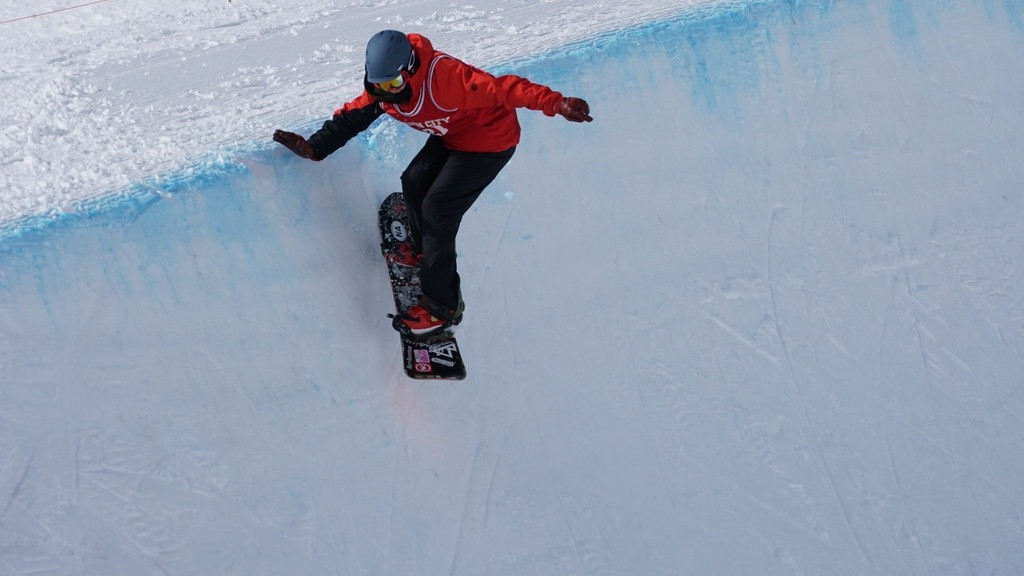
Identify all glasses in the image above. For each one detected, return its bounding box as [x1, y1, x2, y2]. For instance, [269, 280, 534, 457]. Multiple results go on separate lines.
[376, 70, 411, 94]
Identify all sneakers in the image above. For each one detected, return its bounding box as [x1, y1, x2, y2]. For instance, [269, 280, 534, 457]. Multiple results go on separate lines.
[393, 303, 463, 336]
[383, 241, 424, 266]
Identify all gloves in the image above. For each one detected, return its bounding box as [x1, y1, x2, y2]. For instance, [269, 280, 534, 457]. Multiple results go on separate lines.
[274, 129, 319, 161]
[555, 98, 593, 123]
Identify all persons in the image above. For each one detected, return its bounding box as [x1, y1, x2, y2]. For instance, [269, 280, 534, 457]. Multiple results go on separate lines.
[272, 30, 593, 343]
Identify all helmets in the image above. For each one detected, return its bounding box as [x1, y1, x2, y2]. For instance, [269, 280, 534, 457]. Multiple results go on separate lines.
[365, 30, 417, 82]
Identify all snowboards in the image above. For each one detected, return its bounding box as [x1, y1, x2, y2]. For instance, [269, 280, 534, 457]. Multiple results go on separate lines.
[377, 191, 466, 383]
